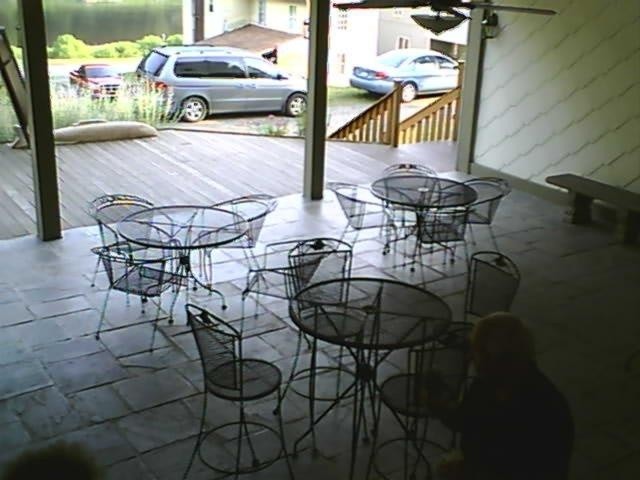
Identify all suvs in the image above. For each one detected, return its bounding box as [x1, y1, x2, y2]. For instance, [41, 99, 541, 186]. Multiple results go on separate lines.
[137, 45, 309, 123]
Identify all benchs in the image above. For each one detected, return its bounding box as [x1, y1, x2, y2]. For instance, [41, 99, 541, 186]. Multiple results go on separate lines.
[546, 174, 640, 245]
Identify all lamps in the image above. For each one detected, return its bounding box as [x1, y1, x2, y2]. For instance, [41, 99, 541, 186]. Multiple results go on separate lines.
[481, 14, 499, 39]
[411, 11, 464, 35]
[304, 22, 311, 37]
[15, 26, 23, 48]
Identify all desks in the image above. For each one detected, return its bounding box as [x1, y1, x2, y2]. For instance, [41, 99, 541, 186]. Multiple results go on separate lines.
[117, 205, 248, 324]
[289, 278, 453, 475]
[370, 175, 477, 273]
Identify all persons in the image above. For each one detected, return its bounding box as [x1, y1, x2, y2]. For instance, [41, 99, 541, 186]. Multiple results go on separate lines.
[1, 439, 106, 479]
[437, 312, 575, 480]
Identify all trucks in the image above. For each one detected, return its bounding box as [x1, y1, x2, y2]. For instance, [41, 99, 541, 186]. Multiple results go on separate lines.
[68, 63, 128, 99]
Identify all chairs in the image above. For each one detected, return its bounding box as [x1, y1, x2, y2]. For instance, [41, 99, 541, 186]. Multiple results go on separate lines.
[411, 208, 475, 288]
[367, 319, 475, 480]
[186, 194, 278, 295]
[442, 176, 511, 256]
[382, 162, 441, 239]
[325, 182, 399, 268]
[405, 251, 522, 429]
[289, 238, 352, 351]
[87, 193, 173, 296]
[91, 248, 185, 352]
[182, 304, 294, 480]
[243, 240, 333, 319]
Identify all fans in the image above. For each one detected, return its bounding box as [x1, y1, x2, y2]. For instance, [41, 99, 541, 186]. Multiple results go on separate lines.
[332, 0, 557, 16]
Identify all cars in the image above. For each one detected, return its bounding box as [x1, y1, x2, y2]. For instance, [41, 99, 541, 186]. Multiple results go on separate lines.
[349, 48, 459, 102]
